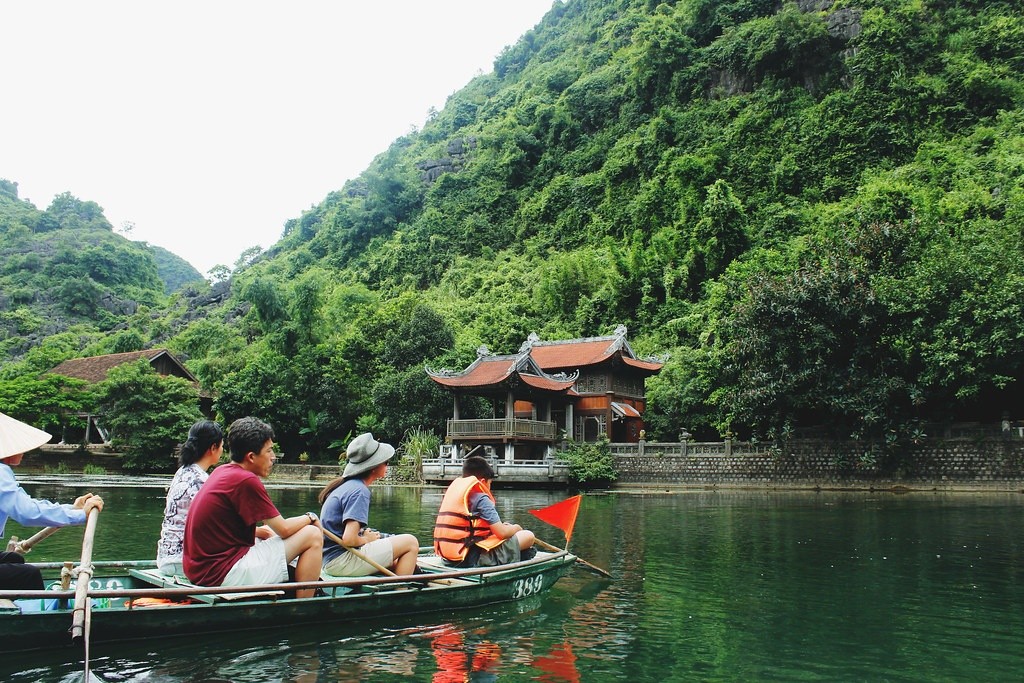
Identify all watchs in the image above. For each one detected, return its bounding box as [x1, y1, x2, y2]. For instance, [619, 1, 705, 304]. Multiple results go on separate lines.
[305, 511, 316, 526]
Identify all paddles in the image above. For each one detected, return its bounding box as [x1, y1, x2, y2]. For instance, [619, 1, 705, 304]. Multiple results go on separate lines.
[534, 535, 613, 580]
[71, 508, 100, 683]
[4, 501, 85, 558]
[319, 525, 397, 578]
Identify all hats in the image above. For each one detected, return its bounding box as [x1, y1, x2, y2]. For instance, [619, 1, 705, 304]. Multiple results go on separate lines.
[0, 412, 52, 459]
[342, 432, 395, 478]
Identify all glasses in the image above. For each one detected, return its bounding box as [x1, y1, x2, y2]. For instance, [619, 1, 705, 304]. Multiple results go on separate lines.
[383, 461, 389, 466]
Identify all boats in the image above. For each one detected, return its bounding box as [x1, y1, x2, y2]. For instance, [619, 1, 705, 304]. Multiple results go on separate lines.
[0, 547, 579, 646]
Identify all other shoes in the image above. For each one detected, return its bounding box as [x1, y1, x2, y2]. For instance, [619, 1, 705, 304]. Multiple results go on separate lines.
[520, 546, 537, 562]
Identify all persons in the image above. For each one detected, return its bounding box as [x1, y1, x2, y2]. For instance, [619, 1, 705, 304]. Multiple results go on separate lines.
[320, 433, 420, 577]
[0, 412, 105, 590]
[433, 457, 537, 568]
[157, 416, 324, 598]
